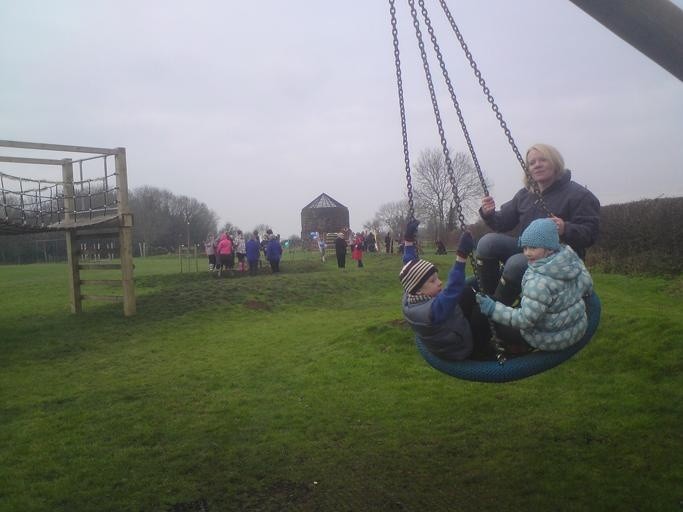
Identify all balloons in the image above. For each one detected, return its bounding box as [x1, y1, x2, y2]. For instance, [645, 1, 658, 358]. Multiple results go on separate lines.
[310, 232, 320, 240]
[284, 241, 289, 249]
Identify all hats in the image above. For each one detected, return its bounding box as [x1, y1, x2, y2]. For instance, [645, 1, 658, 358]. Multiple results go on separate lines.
[396, 258, 440, 295]
[516, 217, 561, 252]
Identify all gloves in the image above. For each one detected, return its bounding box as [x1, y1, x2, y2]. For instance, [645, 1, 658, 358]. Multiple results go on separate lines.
[403, 220, 421, 243]
[474, 291, 496, 318]
[455, 231, 475, 260]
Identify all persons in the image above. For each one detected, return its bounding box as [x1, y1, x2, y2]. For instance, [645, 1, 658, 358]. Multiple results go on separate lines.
[204, 228, 283, 278]
[398, 215, 491, 362]
[471, 142, 600, 296]
[317, 225, 405, 270]
[473, 217, 593, 354]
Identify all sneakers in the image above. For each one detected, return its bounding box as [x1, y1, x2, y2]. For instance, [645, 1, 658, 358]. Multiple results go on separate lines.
[206, 261, 286, 278]
